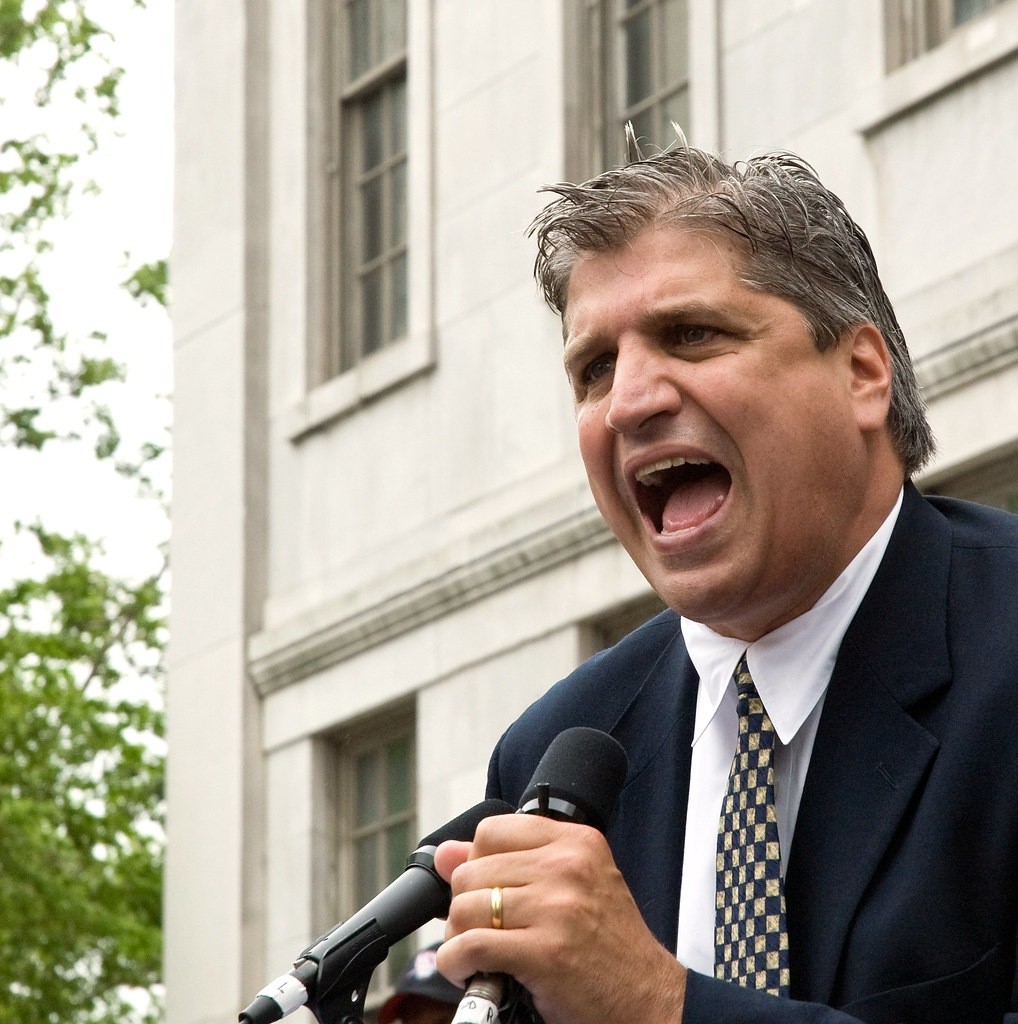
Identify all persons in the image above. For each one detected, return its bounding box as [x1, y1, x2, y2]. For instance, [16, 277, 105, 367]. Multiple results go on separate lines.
[434, 146, 1018, 1024]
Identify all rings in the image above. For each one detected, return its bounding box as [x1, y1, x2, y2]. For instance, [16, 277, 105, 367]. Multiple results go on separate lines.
[492, 887, 503, 928]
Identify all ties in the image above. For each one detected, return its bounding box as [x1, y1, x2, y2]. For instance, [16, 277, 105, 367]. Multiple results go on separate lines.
[714, 653, 790, 1003]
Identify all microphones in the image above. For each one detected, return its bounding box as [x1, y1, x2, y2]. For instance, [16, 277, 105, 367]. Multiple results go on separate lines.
[449, 727, 630, 1024]
[236, 797, 518, 1024]
